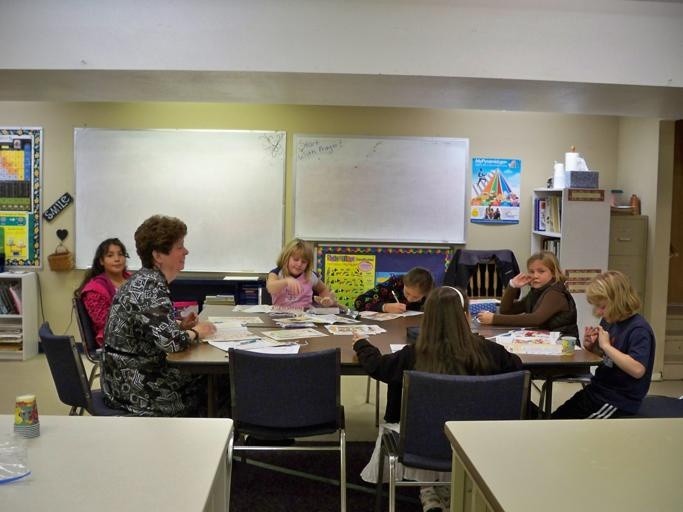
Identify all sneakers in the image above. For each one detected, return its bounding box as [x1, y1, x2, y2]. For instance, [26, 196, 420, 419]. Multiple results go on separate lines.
[435, 486, 450, 507]
[418, 486, 447, 512]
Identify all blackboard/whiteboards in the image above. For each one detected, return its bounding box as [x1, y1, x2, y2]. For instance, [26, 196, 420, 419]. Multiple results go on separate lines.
[73, 126, 287, 275]
[291, 133, 471, 246]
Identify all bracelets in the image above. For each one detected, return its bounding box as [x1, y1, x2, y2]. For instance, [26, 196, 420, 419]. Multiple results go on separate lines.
[509, 279, 517, 289]
[187, 327, 200, 341]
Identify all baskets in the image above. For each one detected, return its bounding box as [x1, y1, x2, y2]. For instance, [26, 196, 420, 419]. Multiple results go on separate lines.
[46, 244, 75, 272]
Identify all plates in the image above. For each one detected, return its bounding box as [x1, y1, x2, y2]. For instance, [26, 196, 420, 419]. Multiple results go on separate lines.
[404, 326, 420, 339]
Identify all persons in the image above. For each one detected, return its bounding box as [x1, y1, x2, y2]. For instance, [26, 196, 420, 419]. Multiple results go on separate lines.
[265, 237, 335, 308]
[551, 271, 655, 418]
[475, 253, 579, 346]
[74, 237, 132, 349]
[354, 267, 433, 313]
[476, 168, 486, 186]
[484, 205, 500, 221]
[351, 286, 521, 511]
[100, 215, 217, 416]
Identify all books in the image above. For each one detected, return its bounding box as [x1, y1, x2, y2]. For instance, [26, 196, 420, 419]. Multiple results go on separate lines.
[0, 318, 21, 350]
[534, 195, 560, 232]
[0, 281, 20, 314]
[202, 287, 258, 310]
[539, 238, 559, 259]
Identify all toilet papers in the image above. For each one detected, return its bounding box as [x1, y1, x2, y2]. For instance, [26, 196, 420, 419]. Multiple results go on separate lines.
[564, 152, 579, 171]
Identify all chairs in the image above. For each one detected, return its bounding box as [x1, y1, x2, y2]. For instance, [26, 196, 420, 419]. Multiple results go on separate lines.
[221, 347, 347, 512]
[445, 249, 519, 314]
[635, 390, 683, 417]
[377, 371, 534, 510]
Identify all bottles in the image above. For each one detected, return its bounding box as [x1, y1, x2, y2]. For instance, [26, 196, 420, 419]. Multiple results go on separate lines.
[630, 193, 640, 216]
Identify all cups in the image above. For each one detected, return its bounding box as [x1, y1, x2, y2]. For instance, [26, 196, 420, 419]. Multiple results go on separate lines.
[560, 337, 576, 357]
[13, 392, 40, 440]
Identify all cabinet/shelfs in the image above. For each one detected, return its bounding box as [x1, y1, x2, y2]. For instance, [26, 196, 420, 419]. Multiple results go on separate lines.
[612, 217, 644, 307]
[531, 188, 608, 278]
[0, 268, 40, 361]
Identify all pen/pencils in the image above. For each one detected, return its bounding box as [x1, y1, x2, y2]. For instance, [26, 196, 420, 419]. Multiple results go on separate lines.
[392, 291, 399, 303]
[241, 340, 256, 344]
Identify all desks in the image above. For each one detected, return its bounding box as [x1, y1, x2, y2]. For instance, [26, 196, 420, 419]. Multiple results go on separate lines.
[3, 413, 232, 510]
[446, 416, 683, 511]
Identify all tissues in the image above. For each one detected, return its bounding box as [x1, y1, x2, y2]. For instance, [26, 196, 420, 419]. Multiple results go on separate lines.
[566, 158, 600, 189]
